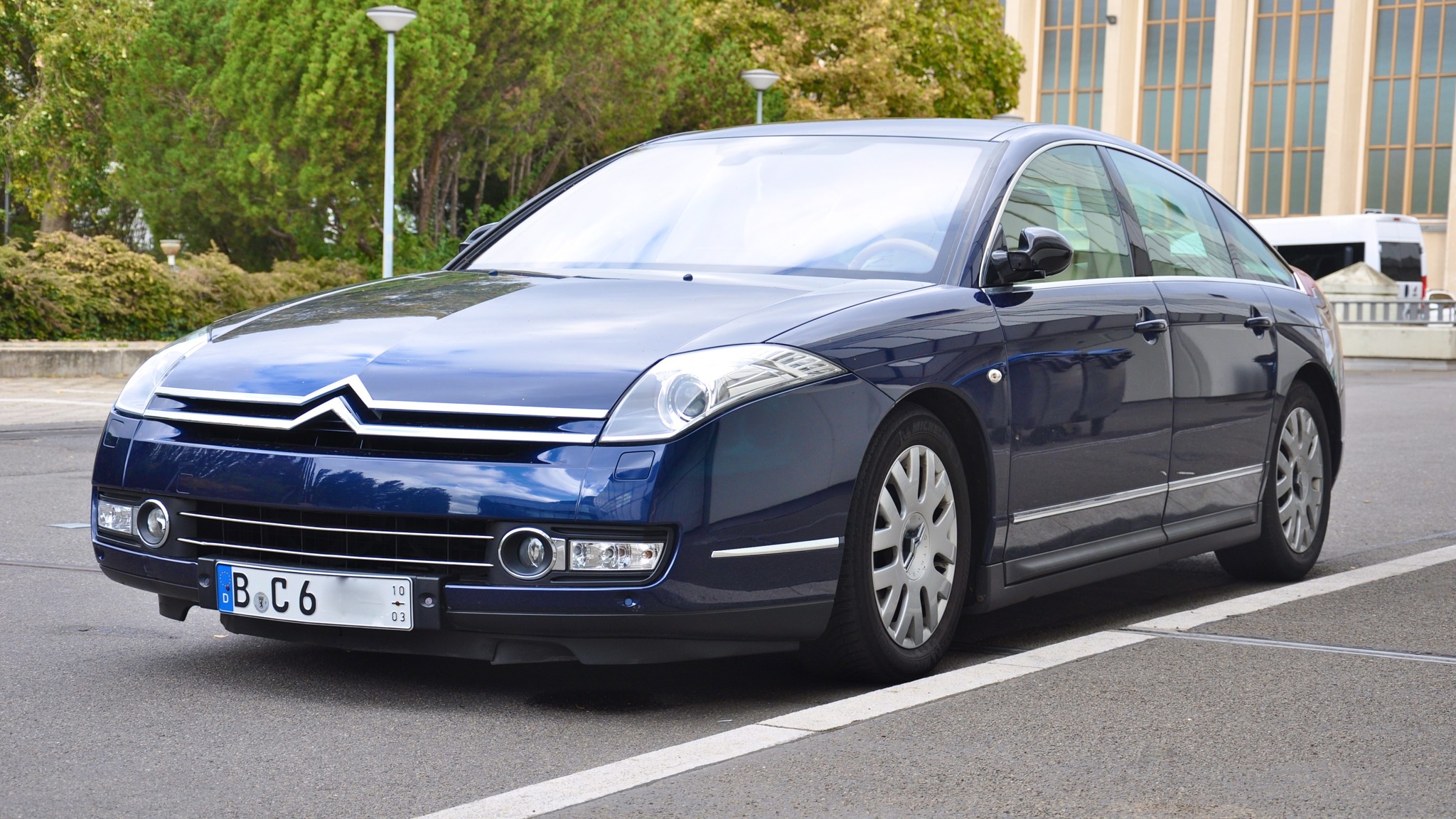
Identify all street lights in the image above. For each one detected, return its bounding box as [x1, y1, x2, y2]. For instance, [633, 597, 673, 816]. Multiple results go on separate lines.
[740, 67, 782, 123]
[365, 5, 419, 280]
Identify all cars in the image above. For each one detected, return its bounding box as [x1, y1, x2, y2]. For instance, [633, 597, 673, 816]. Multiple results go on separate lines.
[88, 118, 1351, 690]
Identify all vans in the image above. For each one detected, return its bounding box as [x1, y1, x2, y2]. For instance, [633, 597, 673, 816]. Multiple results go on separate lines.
[1170, 212, 1431, 326]
